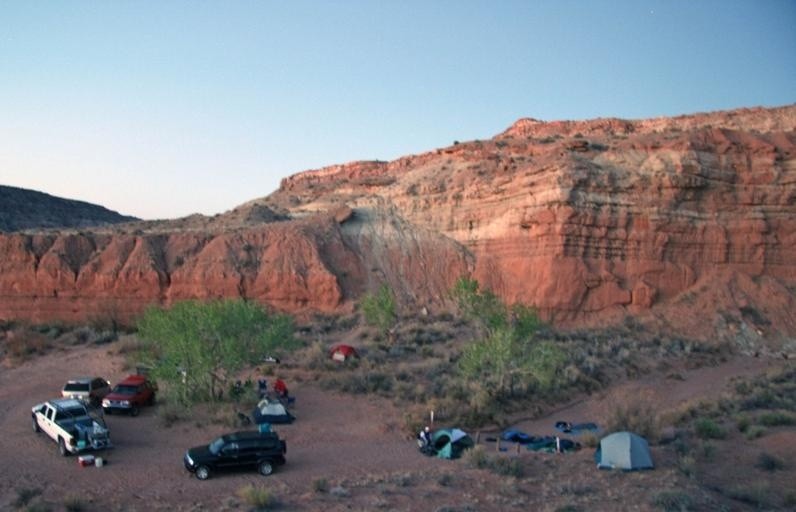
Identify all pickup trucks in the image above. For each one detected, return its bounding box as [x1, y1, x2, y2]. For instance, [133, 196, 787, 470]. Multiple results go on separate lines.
[31, 397, 114, 456]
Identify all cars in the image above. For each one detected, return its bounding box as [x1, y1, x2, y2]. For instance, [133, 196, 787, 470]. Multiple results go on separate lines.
[59, 374, 158, 418]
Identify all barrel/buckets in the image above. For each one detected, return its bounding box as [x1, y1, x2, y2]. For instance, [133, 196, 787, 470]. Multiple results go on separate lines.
[94, 457, 103, 467]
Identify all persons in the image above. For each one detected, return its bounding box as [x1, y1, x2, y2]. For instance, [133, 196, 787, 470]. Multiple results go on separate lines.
[233, 406, 252, 424]
[255, 372, 290, 403]
[417, 424, 433, 451]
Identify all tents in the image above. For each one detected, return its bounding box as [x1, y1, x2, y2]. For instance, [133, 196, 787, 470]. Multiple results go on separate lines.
[428, 427, 476, 461]
[329, 343, 359, 367]
[594, 430, 655, 471]
[252, 393, 295, 425]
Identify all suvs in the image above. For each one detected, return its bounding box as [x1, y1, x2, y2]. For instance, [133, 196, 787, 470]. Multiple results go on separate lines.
[182, 430, 288, 481]
